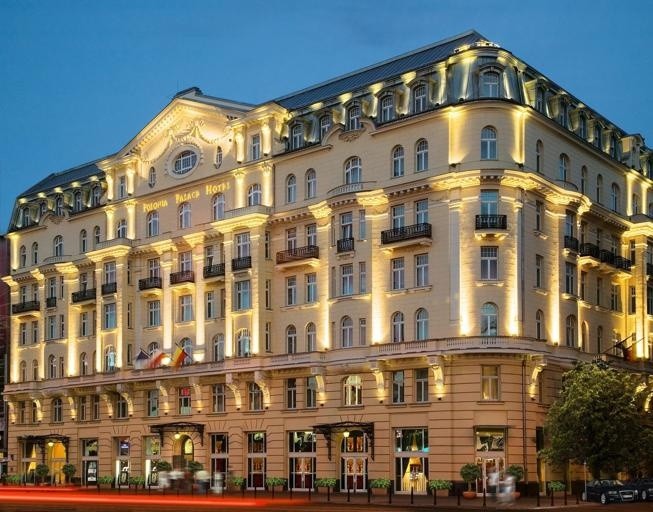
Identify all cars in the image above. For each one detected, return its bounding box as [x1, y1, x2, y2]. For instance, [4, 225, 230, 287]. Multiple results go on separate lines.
[623, 478, 652, 501]
[577, 477, 638, 503]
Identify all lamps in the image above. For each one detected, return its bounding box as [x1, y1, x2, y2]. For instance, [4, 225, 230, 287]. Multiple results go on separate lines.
[342, 427, 350, 438]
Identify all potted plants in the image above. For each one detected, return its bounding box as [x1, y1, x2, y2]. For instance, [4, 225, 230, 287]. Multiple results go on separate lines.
[429, 463, 482, 499]
[224, 476, 389, 497]
[2, 464, 144, 491]
[187, 461, 204, 491]
[506, 465, 570, 501]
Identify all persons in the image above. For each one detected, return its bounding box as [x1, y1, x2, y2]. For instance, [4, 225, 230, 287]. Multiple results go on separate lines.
[155, 466, 225, 497]
[500, 472, 518, 506]
[487, 466, 501, 503]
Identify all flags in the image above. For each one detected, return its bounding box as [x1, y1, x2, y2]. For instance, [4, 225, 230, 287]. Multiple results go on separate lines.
[150, 350, 164, 365]
[132, 351, 148, 371]
[168, 347, 187, 372]
